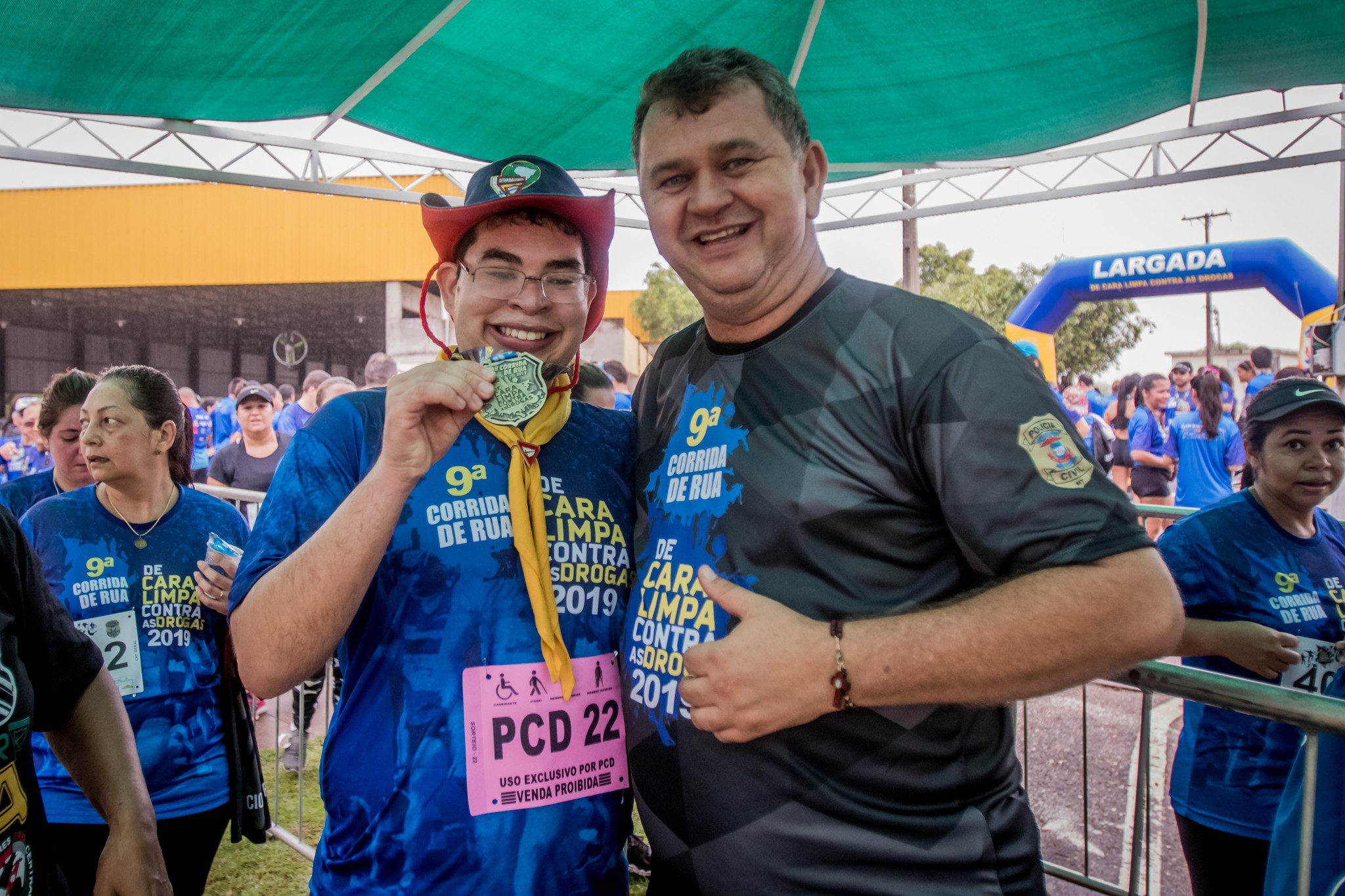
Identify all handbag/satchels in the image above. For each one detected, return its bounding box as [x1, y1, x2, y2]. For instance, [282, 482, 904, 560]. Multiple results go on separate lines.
[1091, 423, 1115, 475]
[217, 607, 272, 844]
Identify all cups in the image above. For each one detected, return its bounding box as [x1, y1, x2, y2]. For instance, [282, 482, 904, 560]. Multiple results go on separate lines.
[202, 542, 243, 599]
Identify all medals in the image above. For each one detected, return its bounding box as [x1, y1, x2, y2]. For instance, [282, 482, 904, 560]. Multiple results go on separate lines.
[472, 352, 548, 426]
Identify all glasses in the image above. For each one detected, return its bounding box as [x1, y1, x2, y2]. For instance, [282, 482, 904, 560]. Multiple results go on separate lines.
[456, 257, 595, 304]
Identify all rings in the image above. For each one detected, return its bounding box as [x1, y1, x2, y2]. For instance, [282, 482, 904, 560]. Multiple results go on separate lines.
[216, 591, 223, 600]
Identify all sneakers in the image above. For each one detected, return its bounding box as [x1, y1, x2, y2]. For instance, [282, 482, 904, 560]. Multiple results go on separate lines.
[283, 731, 310, 776]
[624, 834, 656, 877]
[243, 687, 270, 720]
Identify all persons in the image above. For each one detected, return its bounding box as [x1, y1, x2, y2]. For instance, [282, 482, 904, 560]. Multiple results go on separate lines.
[0, 345, 1317, 895]
[625, 47, 1185, 895]
[227, 155, 640, 895]
[1159, 374, 1345, 895]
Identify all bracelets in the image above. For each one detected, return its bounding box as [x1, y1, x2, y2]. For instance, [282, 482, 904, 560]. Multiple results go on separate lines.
[831, 620, 855, 711]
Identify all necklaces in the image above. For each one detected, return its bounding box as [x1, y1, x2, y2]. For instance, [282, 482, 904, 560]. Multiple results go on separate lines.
[107, 481, 174, 550]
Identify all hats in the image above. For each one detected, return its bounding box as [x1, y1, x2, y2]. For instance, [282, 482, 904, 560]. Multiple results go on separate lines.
[603, 361, 630, 384]
[1170, 363, 1192, 376]
[235, 386, 274, 407]
[419, 155, 616, 342]
[1059, 386, 1091, 411]
[1246, 375, 1345, 422]
